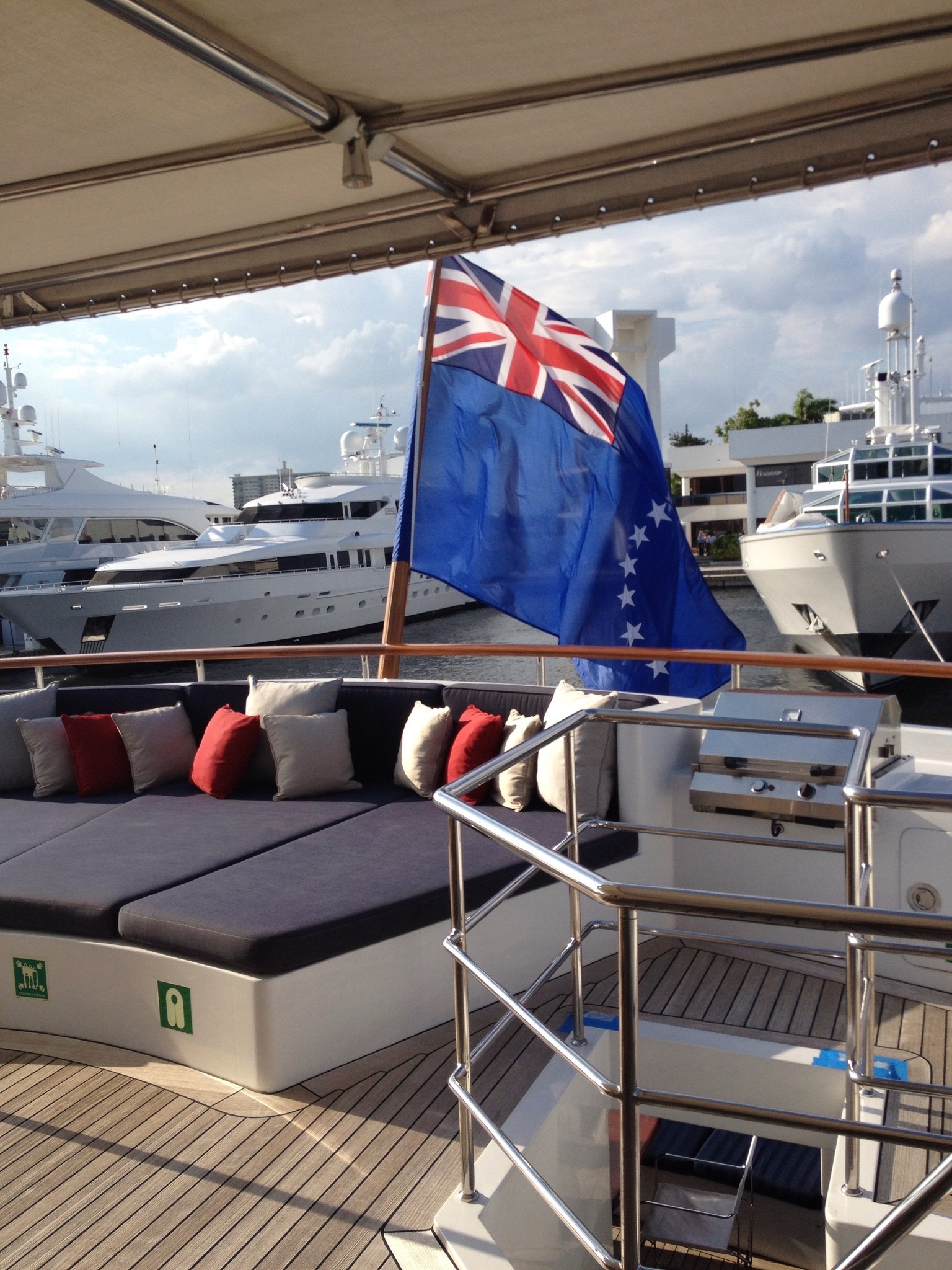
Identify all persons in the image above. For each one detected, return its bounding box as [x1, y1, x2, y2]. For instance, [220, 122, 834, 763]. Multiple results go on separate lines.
[698, 530, 705, 557]
[703, 532, 711, 557]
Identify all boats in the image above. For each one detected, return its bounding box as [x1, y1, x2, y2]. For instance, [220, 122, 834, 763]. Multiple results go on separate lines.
[2, 396, 488, 680]
[3, 338, 240, 673]
[736, 264, 952, 692]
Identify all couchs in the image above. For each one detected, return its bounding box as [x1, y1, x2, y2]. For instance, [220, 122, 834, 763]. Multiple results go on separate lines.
[0, 682, 707, 1088]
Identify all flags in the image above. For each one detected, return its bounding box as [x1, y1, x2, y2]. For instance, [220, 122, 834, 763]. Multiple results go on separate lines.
[388, 253, 747, 701]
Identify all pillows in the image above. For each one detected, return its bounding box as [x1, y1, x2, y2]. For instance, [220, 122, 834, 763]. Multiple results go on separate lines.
[393, 699, 452, 806]
[16, 716, 76, 800]
[441, 704, 503, 802]
[0, 680, 61, 791]
[488, 707, 541, 808]
[108, 702, 200, 794]
[244, 671, 343, 770]
[189, 702, 262, 802]
[261, 708, 368, 805]
[60, 711, 134, 795]
[531, 677, 623, 823]
